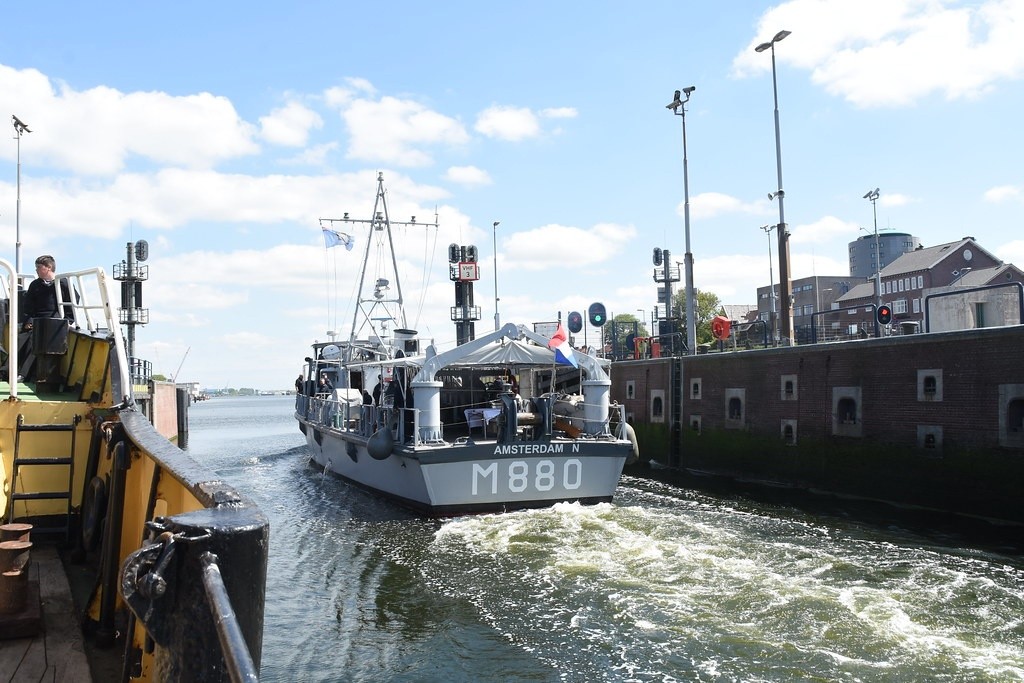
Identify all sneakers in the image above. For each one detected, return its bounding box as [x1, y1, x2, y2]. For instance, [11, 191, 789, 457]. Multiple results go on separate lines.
[16, 374, 25, 383]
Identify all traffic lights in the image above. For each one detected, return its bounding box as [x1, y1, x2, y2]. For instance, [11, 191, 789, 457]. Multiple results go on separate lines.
[589, 302, 606, 327]
[876, 305, 892, 326]
[567, 311, 582, 334]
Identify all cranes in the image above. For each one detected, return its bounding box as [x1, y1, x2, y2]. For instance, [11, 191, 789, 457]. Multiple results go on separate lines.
[169, 345, 191, 383]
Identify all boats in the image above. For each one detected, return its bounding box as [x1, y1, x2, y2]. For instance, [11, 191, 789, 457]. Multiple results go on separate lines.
[5, 256, 267, 683]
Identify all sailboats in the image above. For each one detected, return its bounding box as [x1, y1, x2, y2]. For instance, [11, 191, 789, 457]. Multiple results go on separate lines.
[294, 166, 638, 515]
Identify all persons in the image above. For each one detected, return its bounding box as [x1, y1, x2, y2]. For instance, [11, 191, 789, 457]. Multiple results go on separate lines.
[296, 373, 387, 420]
[18, 255, 80, 396]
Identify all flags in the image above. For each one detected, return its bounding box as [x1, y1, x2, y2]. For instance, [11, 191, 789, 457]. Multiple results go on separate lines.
[323, 227, 356, 252]
[548, 322, 579, 370]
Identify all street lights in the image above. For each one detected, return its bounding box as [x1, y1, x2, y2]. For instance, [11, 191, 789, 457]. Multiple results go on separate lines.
[756, 28, 800, 345]
[492, 219, 502, 331]
